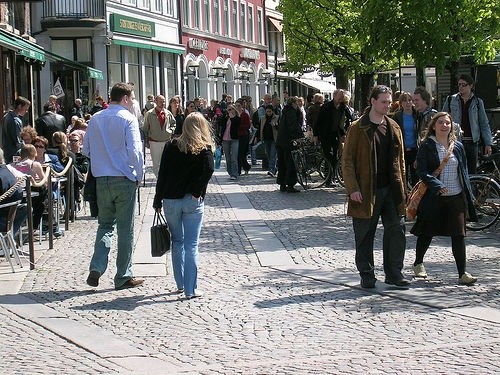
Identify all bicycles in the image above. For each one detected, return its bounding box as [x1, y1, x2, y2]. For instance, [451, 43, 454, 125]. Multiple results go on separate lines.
[289, 130, 346, 190]
[465, 130, 500, 232]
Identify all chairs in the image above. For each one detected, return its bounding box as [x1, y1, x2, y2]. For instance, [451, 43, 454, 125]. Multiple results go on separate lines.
[0, 200, 23, 273]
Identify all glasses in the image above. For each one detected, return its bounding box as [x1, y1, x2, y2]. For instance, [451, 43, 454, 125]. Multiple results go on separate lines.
[228, 109, 232, 111]
[55, 140, 63, 144]
[70, 139, 78, 143]
[34, 145, 43, 149]
[458, 83, 469, 88]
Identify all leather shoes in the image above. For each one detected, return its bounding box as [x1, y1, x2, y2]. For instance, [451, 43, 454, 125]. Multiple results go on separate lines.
[115, 277, 146, 290]
[86, 271, 99, 287]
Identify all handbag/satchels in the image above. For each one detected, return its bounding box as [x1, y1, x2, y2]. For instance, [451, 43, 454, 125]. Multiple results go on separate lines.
[151, 209, 170, 257]
[407, 179, 428, 220]
[214, 146, 222, 169]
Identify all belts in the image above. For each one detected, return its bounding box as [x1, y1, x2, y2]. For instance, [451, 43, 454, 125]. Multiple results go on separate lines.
[462, 141, 473, 144]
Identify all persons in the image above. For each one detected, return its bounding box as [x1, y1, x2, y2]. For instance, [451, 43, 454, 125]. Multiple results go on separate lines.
[411, 111, 478, 285]
[152, 110, 214, 298]
[82, 83, 146, 291]
[393, 87, 437, 196]
[442, 75, 493, 173]
[341, 84, 411, 289]
[0, 95, 110, 256]
[128, 94, 211, 178]
[206, 88, 359, 193]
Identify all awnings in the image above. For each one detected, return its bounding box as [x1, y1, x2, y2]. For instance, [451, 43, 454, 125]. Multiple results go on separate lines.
[0, 32, 46, 62]
[295, 79, 336, 93]
[0, 28, 103, 80]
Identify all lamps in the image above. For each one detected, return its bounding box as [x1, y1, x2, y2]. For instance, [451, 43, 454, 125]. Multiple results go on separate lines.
[234, 67, 254, 84]
[208, 65, 229, 83]
[183, 61, 200, 80]
[257, 69, 271, 85]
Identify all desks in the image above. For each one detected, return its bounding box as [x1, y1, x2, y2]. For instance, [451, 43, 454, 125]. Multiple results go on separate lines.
[31, 178, 67, 240]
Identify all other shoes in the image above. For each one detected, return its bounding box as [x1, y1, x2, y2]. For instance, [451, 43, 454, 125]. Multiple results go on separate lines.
[458, 272, 478, 284]
[178, 289, 184, 293]
[230, 176, 236, 180]
[412, 262, 428, 278]
[280, 187, 288, 191]
[326, 183, 337, 188]
[0, 249, 4, 257]
[54, 228, 62, 237]
[361, 279, 378, 288]
[187, 289, 201, 299]
[267, 171, 277, 178]
[384, 272, 409, 286]
[287, 188, 300, 193]
